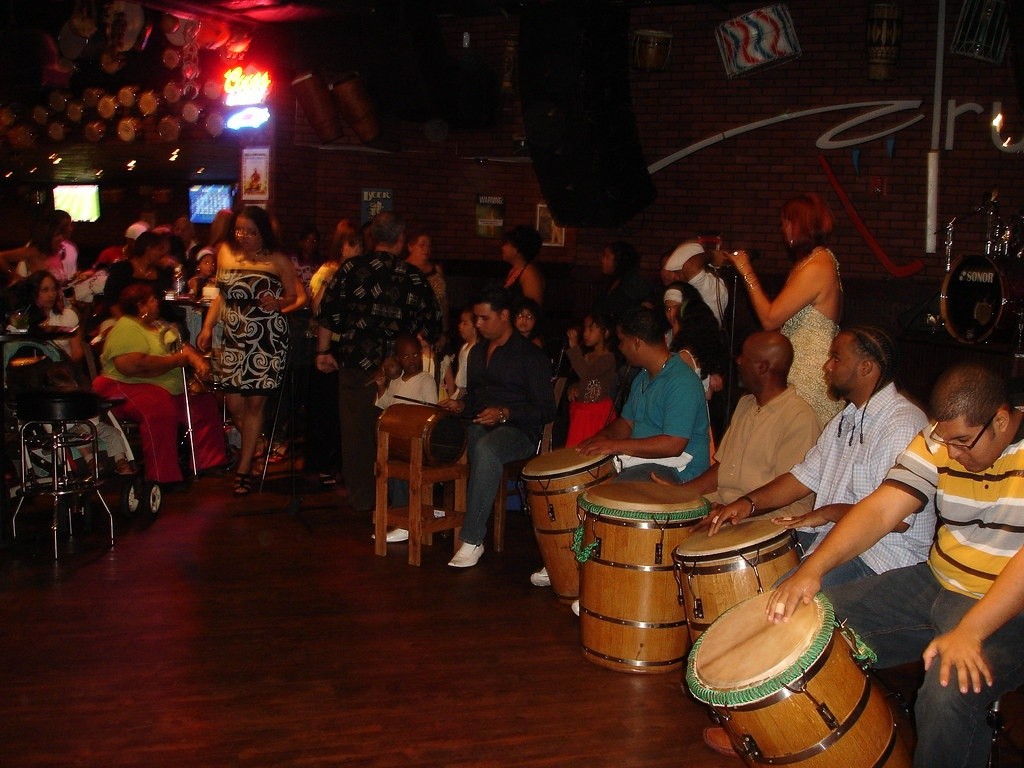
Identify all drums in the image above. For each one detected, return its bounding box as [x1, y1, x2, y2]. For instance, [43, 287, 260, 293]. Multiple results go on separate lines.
[574, 480, 715, 676]
[684, 588, 916, 768]
[672, 517, 803, 650]
[937, 251, 1024, 345]
[374, 402, 467, 467]
[521, 445, 621, 606]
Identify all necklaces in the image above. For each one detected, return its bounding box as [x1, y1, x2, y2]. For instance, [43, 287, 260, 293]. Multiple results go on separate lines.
[39, 313, 56, 329]
[800, 247, 827, 262]
[641, 353, 670, 394]
[233, 247, 263, 264]
[136, 264, 153, 279]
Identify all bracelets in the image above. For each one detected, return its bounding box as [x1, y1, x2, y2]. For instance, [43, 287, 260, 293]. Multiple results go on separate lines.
[737, 260, 760, 294]
[318, 349, 332, 355]
[456, 400, 463, 413]
[738, 496, 756, 517]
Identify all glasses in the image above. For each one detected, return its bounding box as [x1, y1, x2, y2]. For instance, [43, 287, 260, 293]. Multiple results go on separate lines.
[303, 237, 318, 244]
[233, 227, 260, 238]
[929, 409, 1012, 453]
[513, 311, 536, 320]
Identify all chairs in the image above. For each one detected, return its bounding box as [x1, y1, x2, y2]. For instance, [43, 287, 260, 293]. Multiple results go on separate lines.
[11, 391, 114, 562]
[82, 336, 144, 469]
[489, 377, 570, 549]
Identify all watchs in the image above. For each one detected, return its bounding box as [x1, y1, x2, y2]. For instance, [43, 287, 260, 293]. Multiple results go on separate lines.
[499, 408, 506, 424]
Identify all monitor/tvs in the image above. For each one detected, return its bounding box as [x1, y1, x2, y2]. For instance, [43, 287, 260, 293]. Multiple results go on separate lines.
[185, 180, 237, 226]
[52, 183, 102, 226]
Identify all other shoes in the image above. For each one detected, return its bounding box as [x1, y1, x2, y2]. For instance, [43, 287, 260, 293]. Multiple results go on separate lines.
[114, 458, 137, 476]
[56, 456, 79, 473]
[447, 541, 484, 569]
[571, 599, 579, 617]
[703, 726, 740, 758]
[383, 528, 409, 543]
[530, 566, 552, 587]
[144, 481, 191, 495]
[190, 462, 235, 477]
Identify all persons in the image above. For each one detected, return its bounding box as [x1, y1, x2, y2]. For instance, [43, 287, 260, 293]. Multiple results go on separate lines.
[0, 191, 1024, 768]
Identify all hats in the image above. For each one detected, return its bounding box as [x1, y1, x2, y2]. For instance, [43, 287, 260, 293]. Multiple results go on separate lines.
[126, 223, 148, 240]
[663, 243, 704, 272]
[190, 242, 214, 263]
[504, 223, 543, 263]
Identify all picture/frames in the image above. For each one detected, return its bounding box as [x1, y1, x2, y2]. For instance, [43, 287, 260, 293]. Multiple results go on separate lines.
[534, 204, 565, 247]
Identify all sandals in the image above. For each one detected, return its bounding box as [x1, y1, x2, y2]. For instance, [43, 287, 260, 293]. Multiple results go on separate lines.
[233, 469, 252, 495]
[252, 435, 271, 458]
[269, 440, 302, 464]
[319, 472, 338, 489]
